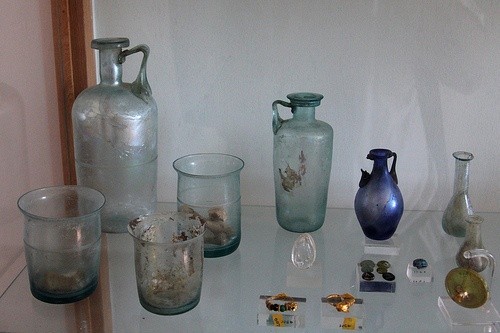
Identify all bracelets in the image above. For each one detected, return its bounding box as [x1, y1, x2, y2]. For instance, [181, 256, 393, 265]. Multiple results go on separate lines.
[326, 294, 355, 312]
[266, 293, 298, 313]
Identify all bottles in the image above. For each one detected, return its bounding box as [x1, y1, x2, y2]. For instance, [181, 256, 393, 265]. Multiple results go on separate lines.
[354, 148, 404, 240]
[442, 150, 475, 238]
[455, 214, 488, 272]
[272, 92, 333, 232]
[71, 37, 158, 232]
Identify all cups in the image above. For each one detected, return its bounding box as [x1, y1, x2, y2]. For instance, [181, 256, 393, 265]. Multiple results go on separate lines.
[126, 209, 207, 315]
[17, 185, 106, 305]
[173, 153, 245, 258]
